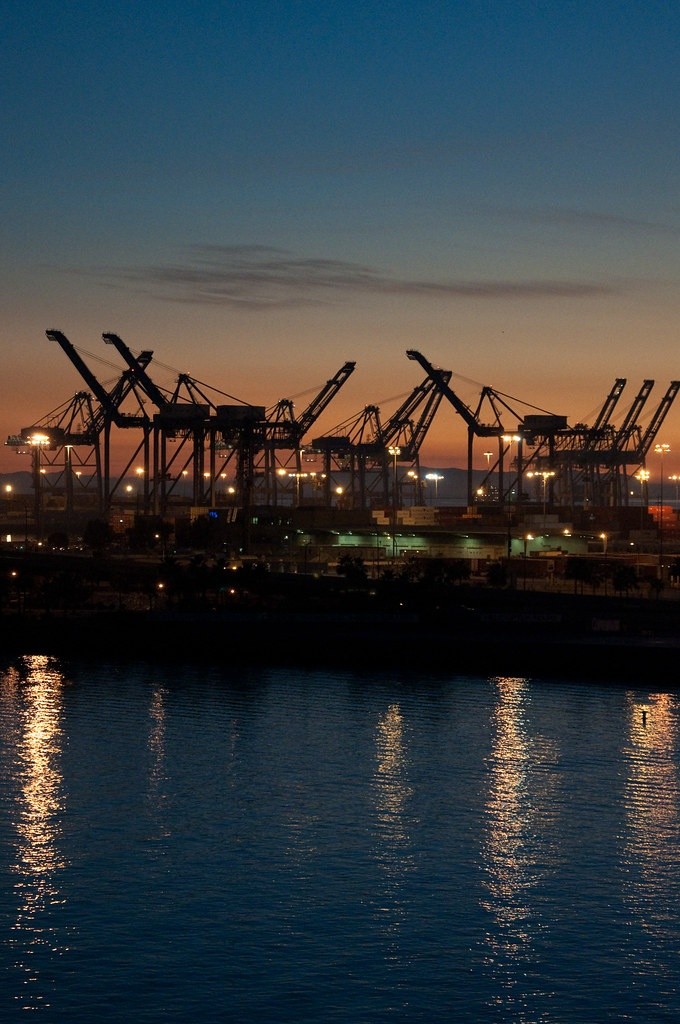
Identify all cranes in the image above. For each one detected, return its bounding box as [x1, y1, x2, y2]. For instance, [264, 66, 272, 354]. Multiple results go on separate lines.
[5, 329, 680, 543]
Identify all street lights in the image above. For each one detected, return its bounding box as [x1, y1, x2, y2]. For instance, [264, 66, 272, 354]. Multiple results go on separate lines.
[670, 474, 680, 523]
[135, 467, 144, 516]
[538, 471, 556, 538]
[634, 469, 651, 530]
[429, 475, 444, 506]
[501, 434, 522, 558]
[653, 444, 672, 579]
[181, 470, 189, 498]
[64, 444, 74, 468]
[387, 446, 400, 537]
